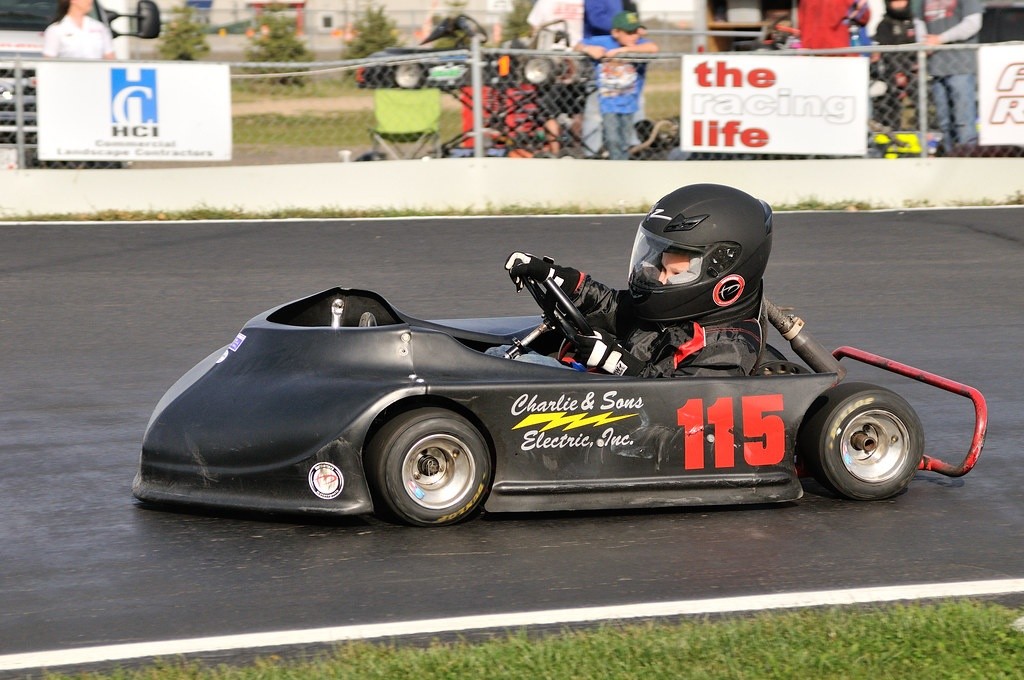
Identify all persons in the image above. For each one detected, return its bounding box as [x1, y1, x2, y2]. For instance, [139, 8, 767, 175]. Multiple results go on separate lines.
[42, 0, 126, 169]
[583, 1, 647, 157]
[527, 1, 584, 154]
[796, 0, 930, 132]
[483, 183, 772, 379]
[573, 11, 655, 160]
[921, 0, 983, 156]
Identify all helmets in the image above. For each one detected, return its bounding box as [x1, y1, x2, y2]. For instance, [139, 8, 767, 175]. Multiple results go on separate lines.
[628, 185, 772, 331]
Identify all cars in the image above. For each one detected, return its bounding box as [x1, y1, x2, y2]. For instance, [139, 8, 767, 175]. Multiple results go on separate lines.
[1, 1, 162, 146]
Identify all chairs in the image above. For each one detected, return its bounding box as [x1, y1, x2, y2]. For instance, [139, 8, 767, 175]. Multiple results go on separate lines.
[369, 88, 444, 161]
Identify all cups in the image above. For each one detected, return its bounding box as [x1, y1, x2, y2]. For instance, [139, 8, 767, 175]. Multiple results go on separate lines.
[338, 150, 352, 162]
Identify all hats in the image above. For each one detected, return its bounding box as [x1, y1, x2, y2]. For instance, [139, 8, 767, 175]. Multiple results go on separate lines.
[612, 11, 647, 32]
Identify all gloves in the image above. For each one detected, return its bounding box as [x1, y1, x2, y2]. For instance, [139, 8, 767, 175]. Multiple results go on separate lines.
[504, 251, 580, 298]
[574, 327, 646, 377]
